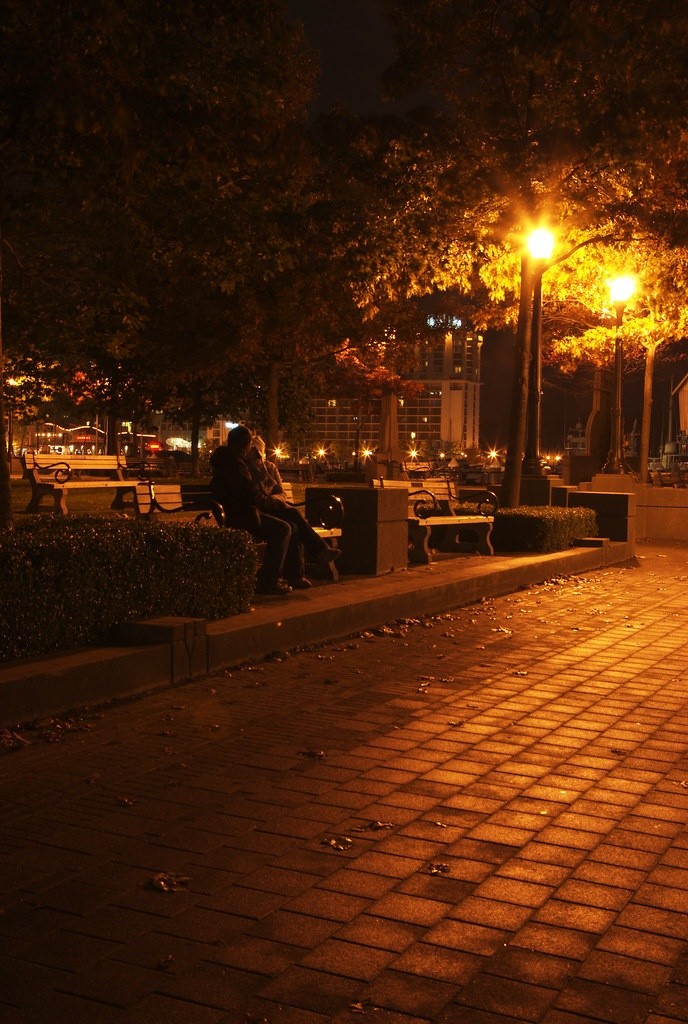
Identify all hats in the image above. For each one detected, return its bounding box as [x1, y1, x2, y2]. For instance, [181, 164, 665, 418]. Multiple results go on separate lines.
[252, 436, 266, 463]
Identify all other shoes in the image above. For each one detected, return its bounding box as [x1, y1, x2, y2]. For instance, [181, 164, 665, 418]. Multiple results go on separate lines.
[316, 549, 342, 563]
[256, 575, 292, 593]
[288, 578, 312, 588]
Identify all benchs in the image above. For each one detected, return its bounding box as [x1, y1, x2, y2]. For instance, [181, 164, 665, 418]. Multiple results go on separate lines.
[370, 477, 500, 564]
[399, 461, 456, 482]
[133, 481, 343, 582]
[21, 449, 152, 516]
[125, 456, 192, 479]
[178, 462, 213, 477]
[279, 464, 317, 478]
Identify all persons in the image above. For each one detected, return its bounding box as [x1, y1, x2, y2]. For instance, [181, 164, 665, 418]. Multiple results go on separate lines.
[209, 424, 341, 592]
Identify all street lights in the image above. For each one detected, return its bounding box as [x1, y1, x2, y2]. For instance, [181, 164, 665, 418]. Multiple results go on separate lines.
[602, 275, 634, 473]
[525, 227, 551, 477]
